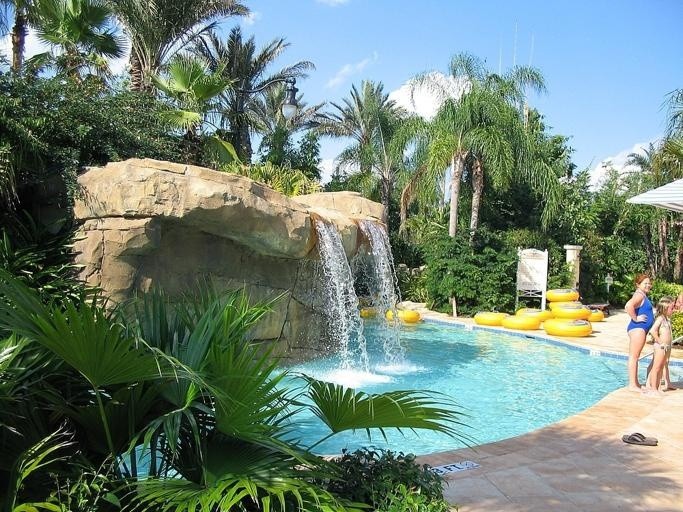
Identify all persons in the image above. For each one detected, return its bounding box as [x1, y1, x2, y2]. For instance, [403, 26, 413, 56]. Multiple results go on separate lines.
[646, 295, 678, 397]
[623, 271, 655, 394]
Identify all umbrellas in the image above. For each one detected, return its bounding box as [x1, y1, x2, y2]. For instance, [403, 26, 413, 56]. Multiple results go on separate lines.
[625, 177, 682, 214]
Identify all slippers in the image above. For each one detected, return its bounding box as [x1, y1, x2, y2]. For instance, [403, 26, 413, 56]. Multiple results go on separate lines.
[621, 433, 658, 446]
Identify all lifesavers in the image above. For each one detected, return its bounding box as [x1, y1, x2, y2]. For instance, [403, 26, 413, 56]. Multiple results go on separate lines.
[361, 307, 420, 324]
[474, 287, 604, 337]
